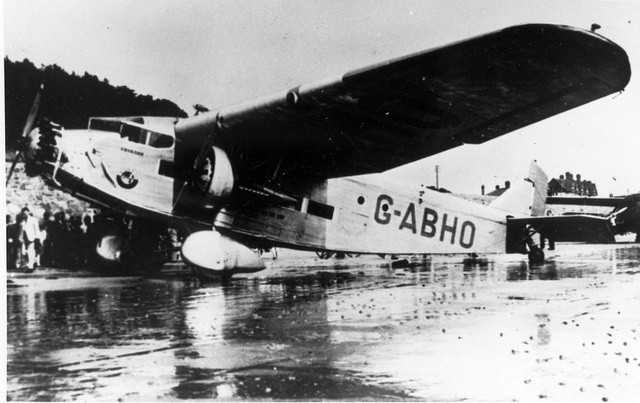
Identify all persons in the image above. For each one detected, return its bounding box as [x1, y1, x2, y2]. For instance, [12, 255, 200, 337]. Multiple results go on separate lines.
[6, 207, 102, 271]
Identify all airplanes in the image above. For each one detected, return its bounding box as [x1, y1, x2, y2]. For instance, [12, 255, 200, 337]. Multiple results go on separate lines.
[6, 21, 640, 280]
